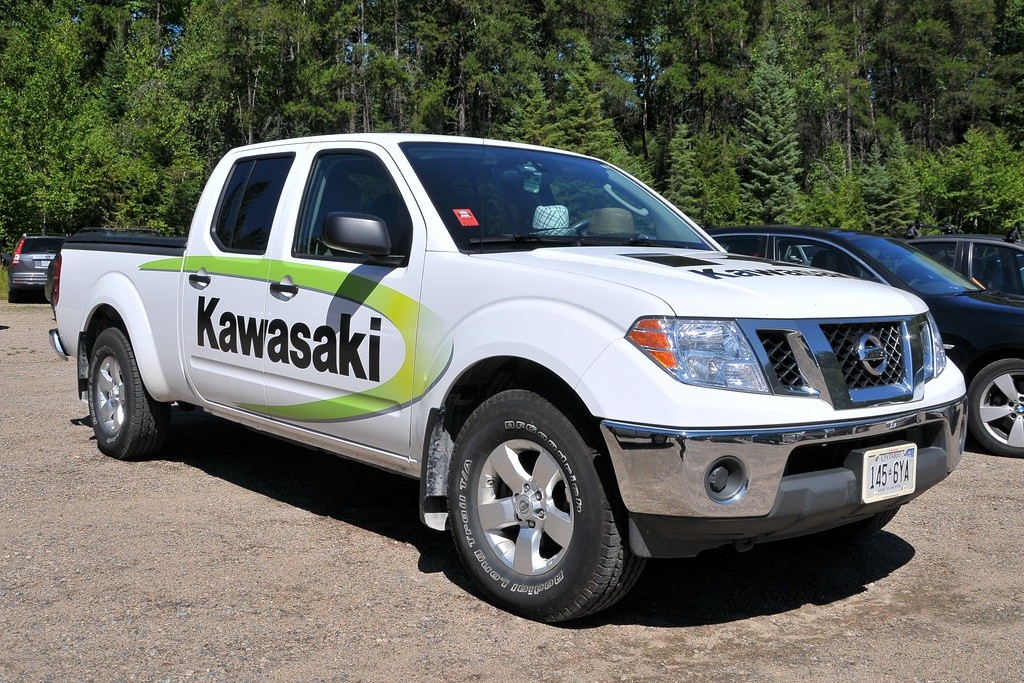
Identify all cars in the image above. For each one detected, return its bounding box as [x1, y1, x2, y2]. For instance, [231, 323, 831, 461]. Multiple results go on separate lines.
[702, 225, 1024, 462]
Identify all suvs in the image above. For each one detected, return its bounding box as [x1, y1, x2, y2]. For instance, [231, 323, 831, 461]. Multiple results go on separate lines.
[1, 231, 71, 303]
[894, 221, 1024, 310]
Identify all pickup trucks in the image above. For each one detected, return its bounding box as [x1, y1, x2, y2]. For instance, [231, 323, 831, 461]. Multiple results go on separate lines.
[47, 132, 970, 617]
